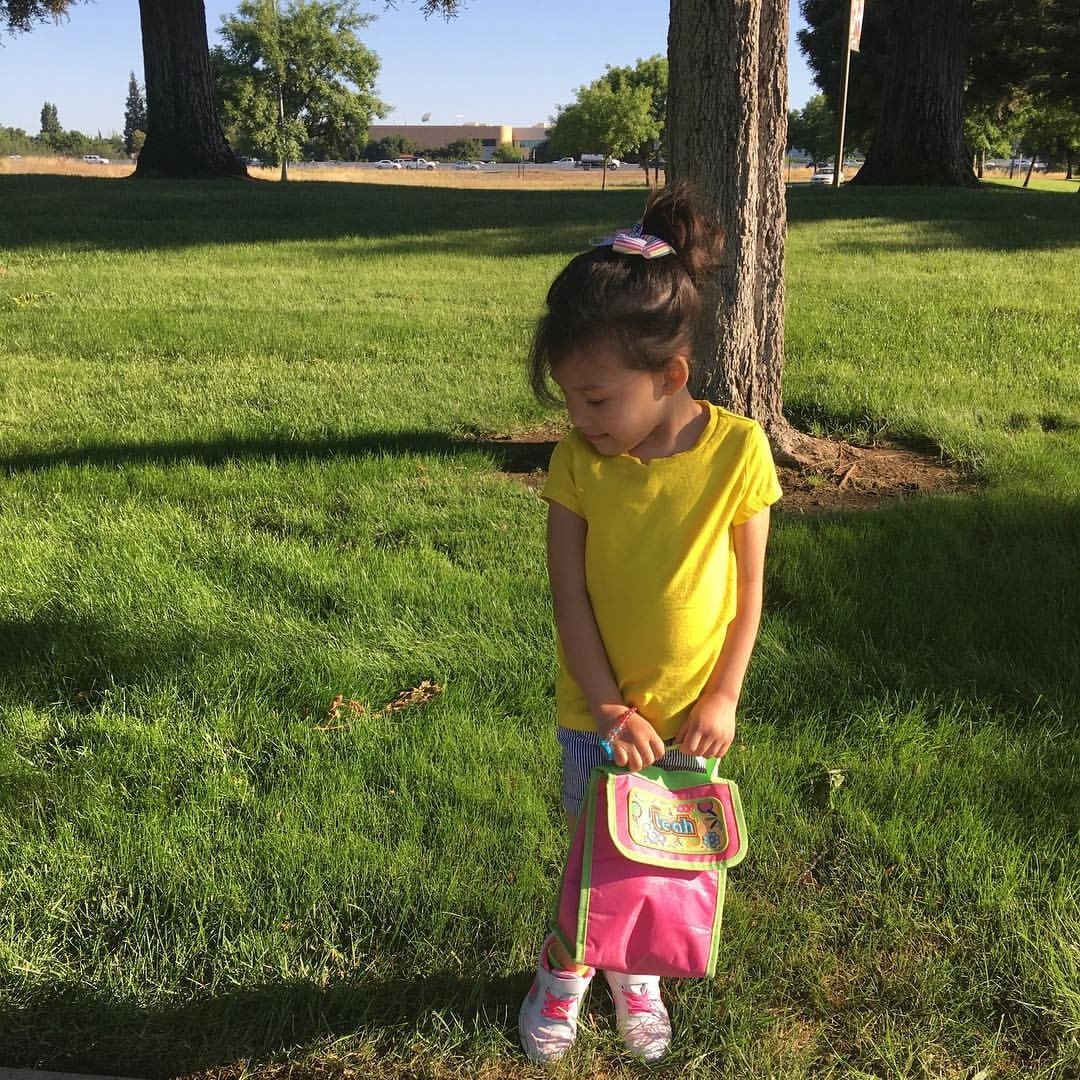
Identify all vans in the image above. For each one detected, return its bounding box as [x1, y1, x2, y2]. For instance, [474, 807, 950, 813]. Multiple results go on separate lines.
[560, 157, 574, 165]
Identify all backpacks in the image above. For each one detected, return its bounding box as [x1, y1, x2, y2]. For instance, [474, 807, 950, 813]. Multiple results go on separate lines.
[547, 741, 750, 979]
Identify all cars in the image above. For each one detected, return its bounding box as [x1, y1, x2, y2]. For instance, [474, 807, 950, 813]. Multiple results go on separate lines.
[82, 155, 109, 165]
[810, 168, 845, 185]
[374, 160, 402, 170]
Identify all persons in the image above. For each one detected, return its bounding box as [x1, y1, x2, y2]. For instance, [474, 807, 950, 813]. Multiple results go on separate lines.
[519, 181, 786, 1066]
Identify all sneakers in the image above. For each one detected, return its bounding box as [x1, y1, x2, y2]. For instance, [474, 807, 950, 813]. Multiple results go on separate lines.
[602, 969, 670, 1064]
[518, 931, 595, 1066]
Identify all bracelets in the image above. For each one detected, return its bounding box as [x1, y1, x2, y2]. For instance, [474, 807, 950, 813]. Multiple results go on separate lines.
[597, 706, 635, 760]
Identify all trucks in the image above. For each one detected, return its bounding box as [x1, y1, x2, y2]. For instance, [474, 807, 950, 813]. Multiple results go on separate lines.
[582, 154, 621, 171]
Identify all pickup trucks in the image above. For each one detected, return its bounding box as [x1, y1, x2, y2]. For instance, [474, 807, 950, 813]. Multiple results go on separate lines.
[403, 159, 436, 170]
[455, 161, 479, 170]
